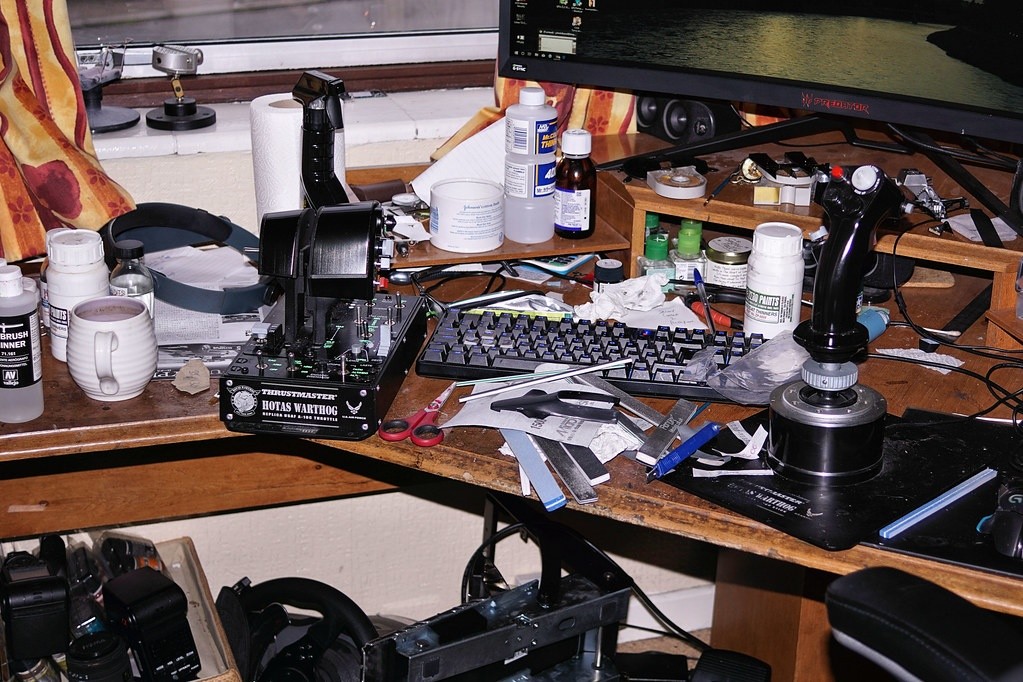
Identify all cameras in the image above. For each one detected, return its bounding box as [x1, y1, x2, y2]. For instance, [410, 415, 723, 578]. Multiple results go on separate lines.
[1, 550, 70, 657]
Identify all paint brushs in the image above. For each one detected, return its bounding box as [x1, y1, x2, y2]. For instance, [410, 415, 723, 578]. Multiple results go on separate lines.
[702, 171, 734, 207]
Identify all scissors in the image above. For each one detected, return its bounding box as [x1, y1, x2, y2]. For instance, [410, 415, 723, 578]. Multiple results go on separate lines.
[378, 381, 457, 448]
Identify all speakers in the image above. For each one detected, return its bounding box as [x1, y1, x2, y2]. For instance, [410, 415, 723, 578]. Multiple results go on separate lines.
[637, 96, 742, 147]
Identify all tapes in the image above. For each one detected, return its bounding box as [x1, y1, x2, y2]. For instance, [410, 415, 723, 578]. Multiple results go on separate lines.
[756, 160, 818, 185]
[646, 165, 707, 199]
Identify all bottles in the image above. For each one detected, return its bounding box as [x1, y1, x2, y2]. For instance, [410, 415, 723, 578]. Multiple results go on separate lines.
[642, 213, 705, 295]
[556, 129, 597, 237]
[0, 258, 50, 424]
[109, 239, 155, 328]
[501, 88, 559, 245]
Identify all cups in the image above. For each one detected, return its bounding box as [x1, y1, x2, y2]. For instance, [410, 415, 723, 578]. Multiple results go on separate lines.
[69, 297, 158, 403]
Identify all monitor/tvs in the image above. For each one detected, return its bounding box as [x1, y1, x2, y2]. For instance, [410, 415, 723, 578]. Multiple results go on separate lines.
[498, 0, 1023, 145]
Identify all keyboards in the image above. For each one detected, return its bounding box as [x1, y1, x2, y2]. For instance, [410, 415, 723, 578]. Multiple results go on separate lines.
[414, 308, 773, 408]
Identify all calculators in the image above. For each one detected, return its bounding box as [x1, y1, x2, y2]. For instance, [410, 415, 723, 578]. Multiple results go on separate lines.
[516, 253, 594, 275]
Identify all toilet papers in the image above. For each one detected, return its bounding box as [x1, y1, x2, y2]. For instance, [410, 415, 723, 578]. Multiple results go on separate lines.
[248, 90, 351, 246]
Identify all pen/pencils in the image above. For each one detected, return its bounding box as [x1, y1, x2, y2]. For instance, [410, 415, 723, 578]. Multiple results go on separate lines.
[693, 268, 716, 337]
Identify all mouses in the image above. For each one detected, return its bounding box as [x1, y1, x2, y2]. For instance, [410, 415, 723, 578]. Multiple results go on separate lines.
[992, 489, 1023, 559]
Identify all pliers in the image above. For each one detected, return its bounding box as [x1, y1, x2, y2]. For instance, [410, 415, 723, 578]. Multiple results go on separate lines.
[669, 286, 747, 331]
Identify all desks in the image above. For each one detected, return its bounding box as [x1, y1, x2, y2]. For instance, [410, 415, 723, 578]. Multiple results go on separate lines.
[295, 116, 1023, 682]
[0, 258, 403, 546]
